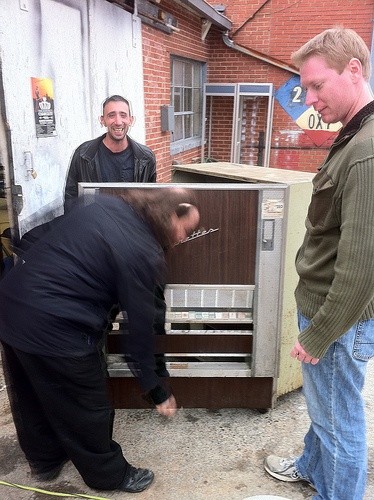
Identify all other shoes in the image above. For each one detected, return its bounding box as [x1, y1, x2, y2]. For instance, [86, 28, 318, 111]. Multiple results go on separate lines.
[40, 459, 70, 481]
[121, 465, 154, 492]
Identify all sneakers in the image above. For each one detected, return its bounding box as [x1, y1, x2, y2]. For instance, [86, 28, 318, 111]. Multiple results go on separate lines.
[263, 455, 318, 490]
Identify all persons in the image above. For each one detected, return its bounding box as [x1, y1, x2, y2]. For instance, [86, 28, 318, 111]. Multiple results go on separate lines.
[263, 27, 372, 499]
[63, 95, 158, 214]
[1, 186, 201, 495]
